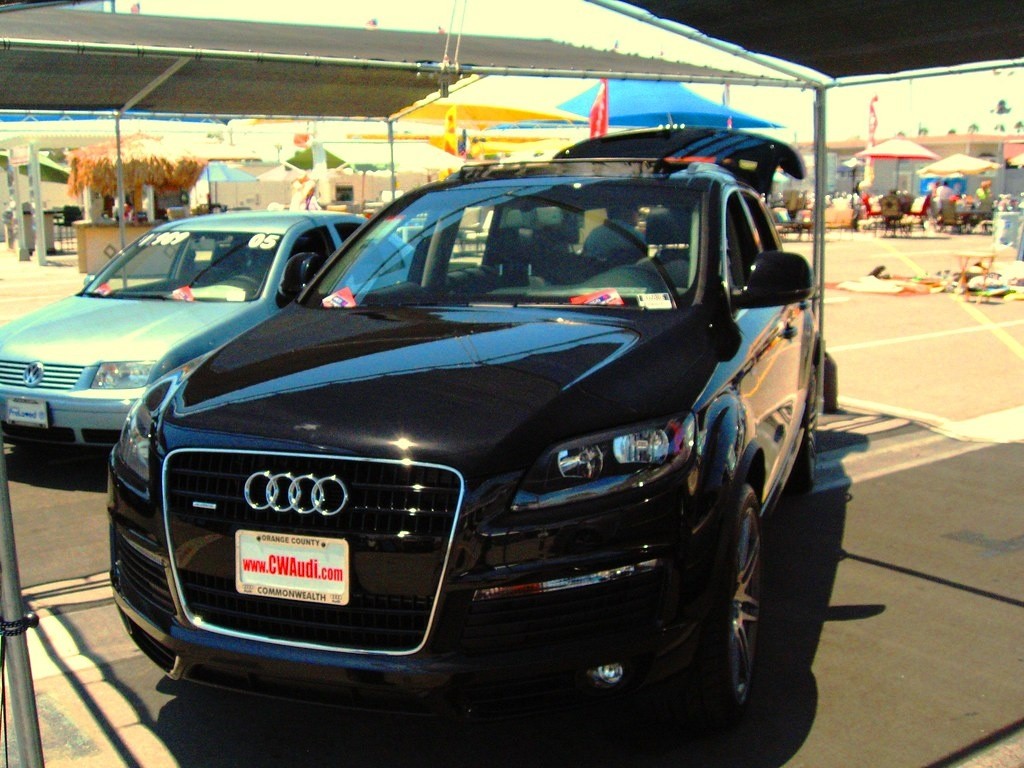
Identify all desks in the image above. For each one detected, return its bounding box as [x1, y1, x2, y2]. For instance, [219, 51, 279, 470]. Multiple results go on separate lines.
[954, 252, 999, 304]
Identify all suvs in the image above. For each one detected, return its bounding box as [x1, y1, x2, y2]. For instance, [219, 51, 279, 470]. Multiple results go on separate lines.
[105, 129, 819, 742]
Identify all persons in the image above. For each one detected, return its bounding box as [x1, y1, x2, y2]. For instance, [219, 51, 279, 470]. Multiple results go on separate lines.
[976, 181, 1024, 212]
[825, 191, 882, 213]
[937, 182, 953, 231]
[124, 203, 136, 219]
[582, 204, 648, 265]
[211, 205, 222, 214]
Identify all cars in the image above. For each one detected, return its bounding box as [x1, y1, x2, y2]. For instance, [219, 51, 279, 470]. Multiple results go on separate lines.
[0, 213, 368, 449]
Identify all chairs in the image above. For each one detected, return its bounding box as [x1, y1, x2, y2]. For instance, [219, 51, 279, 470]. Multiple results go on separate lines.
[772, 206, 813, 240]
[452, 205, 691, 298]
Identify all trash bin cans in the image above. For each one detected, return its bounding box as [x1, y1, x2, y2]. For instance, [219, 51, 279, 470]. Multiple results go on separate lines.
[995, 211, 1020, 246]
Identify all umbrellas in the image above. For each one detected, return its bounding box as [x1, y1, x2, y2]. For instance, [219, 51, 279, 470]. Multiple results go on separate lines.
[71, 133, 210, 210]
[916, 154, 1002, 179]
[856, 133, 941, 190]
[196, 163, 259, 204]
[1009, 153, 1024, 167]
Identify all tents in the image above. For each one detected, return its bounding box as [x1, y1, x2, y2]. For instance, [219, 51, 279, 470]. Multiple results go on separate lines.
[258, 139, 464, 208]
[348, 77, 785, 156]
[0, 0, 826, 389]
